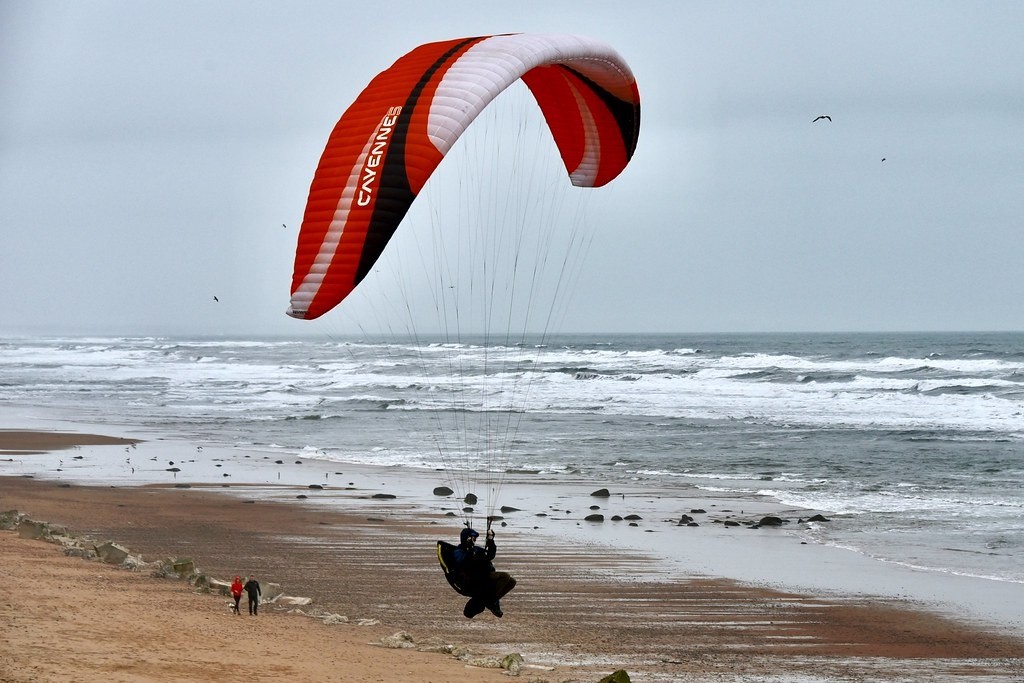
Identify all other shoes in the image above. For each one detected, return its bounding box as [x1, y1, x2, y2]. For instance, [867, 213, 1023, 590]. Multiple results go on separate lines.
[254, 612, 257, 616]
[464, 601, 475, 619]
[487, 601, 503, 618]
[250, 613, 252, 615]
[238, 611, 241, 615]
[233, 610, 236, 614]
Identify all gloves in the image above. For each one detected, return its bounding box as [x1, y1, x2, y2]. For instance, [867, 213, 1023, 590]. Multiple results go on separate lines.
[487, 529, 495, 539]
[467, 536, 476, 547]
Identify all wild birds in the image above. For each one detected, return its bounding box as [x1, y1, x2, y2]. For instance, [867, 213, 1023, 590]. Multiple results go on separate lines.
[283, 223, 286, 229]
[213, 296, 218, 302]
[813, 116, 832, 122]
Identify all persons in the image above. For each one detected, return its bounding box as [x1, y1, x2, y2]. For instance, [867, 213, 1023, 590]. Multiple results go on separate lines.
[453, 528, 517, 618]
[232, 577, 244, 615]
[244, 574, 261, 616]
